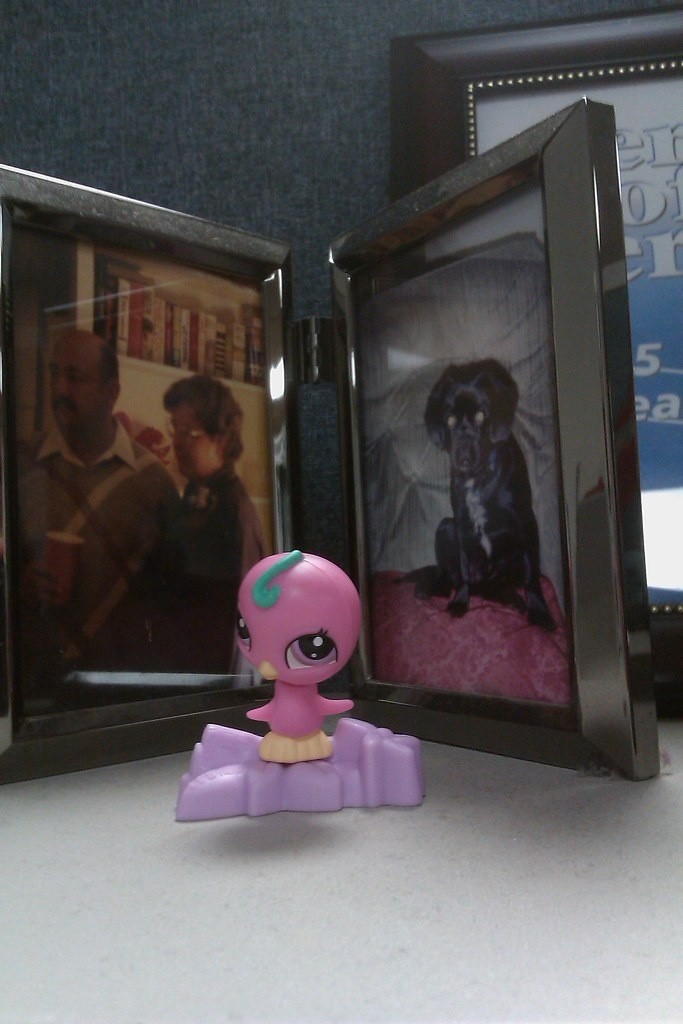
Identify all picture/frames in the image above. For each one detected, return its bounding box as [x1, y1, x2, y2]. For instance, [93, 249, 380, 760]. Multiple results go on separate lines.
[328, 99, 658, 784]
[0, 163, 291, 786]
[389, 2, 683, 724]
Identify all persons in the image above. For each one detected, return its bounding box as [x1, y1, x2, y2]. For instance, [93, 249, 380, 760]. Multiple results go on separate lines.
[128, 373, 266, 683]
[15, 329, 180, 702]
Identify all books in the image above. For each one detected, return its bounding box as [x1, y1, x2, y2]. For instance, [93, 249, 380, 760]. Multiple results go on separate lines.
[89, 268, 265, 383]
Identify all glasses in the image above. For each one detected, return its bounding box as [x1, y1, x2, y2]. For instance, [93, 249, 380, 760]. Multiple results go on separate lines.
[166, 424, 206, 444]
